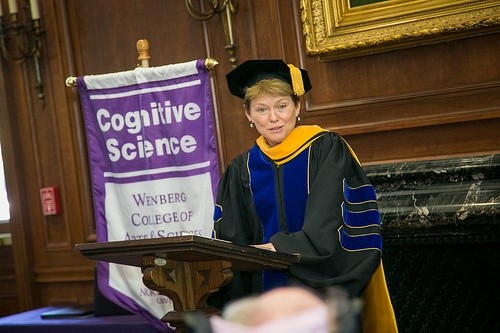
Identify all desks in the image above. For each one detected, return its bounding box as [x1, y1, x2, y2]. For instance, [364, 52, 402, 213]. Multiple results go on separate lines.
[0, 305, 222, 333]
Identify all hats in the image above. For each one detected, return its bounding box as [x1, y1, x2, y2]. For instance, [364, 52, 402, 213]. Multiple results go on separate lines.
[226, 60, 311, 99]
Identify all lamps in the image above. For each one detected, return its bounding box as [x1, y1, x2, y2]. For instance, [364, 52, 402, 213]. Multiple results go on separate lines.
[0, 0, 46, 111]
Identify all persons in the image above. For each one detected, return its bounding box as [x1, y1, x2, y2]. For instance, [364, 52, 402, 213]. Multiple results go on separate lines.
[208, 59, 398, 333]
[208, 286, 335, 333]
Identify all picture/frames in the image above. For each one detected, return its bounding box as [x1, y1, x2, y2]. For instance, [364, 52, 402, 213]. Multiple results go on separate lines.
[300, 0, 500, 62]
